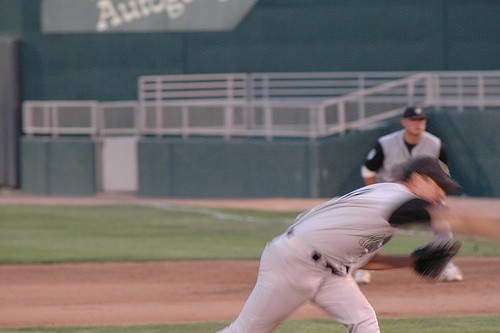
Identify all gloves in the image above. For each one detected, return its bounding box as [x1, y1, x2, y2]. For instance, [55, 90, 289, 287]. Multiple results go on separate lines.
[412, 240, 460, 277]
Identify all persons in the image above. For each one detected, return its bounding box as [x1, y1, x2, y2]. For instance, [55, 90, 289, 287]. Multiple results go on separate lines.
[215, 155, 500, 333]
[354, 107, 463, 283]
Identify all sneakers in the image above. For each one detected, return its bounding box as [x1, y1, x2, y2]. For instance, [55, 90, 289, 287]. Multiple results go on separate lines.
[440, 260, 462, 280]
[354, 268, 370, 283]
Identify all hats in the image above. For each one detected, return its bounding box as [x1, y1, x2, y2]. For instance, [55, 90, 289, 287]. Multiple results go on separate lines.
[409, 157, 463, 196]
[403, 107, 425, 118]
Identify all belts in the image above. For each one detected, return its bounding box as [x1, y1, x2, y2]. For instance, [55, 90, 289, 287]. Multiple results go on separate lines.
[287, 231, 348, 274]
